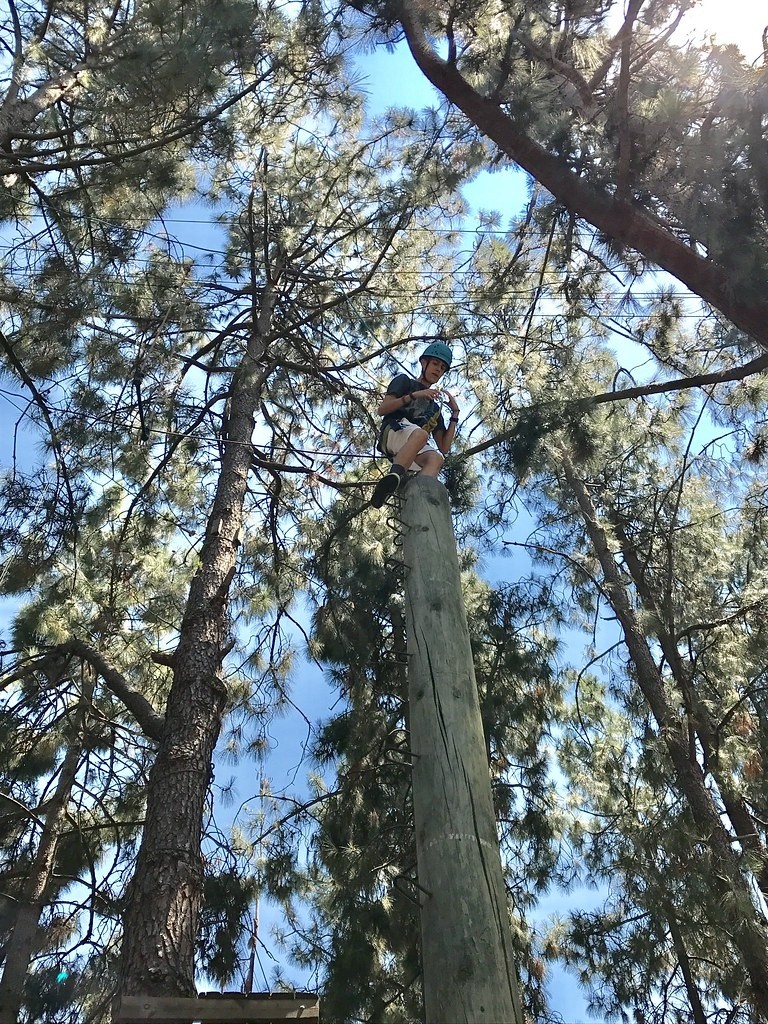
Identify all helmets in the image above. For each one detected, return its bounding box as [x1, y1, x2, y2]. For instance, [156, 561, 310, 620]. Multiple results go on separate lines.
[419, 342, 452, 372]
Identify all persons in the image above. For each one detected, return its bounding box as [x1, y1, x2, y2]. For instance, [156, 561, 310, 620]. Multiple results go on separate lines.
[370, 342, 460, 509]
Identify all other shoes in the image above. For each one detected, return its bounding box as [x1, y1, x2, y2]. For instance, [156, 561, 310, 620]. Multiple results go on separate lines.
[370, 472, 400, 509]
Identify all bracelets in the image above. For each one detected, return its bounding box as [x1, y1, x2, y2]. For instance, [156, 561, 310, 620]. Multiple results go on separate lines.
[408, 392, 416, 400]
[451, 410, 460, 411]
[402, 396, 409, 407]
[450, 417, 458, 422]
